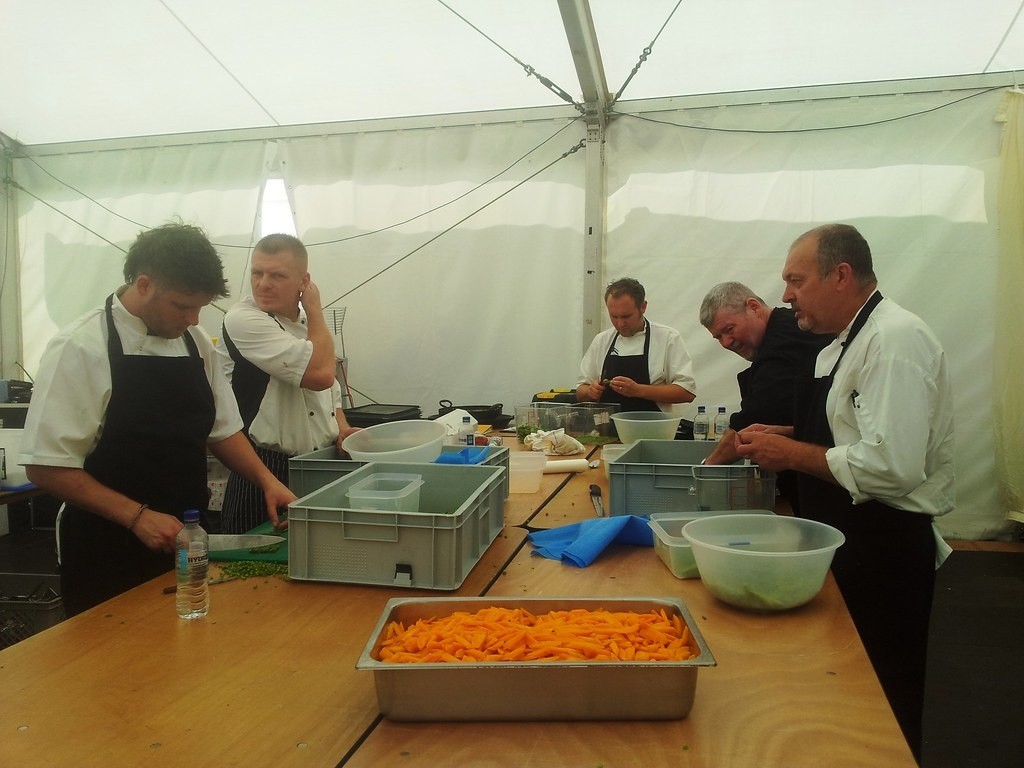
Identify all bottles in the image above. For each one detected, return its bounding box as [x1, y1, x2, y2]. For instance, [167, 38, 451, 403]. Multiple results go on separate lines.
[692, 405, 709, 440]
[174, 510, 211, 620]
[458, 417, 475, 447]
[713, 406, 729, 442]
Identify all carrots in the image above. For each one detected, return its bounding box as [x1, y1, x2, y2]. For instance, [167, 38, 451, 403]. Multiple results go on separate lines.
[378, 606, 695, 662]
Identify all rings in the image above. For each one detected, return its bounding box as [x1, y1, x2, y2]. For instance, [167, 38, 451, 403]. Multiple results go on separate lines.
[619, 387, 622, 393]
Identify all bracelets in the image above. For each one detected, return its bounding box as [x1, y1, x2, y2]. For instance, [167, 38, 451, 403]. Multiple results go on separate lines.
[126, 503, 149, 534]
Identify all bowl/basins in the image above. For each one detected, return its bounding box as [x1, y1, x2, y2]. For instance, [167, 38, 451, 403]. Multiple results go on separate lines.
[647, 509, 779, 580]
[600, 444, 631, 480]
[680, 514, 846, 612]
[341, 419, 447, 464]
[509, 451, 546, 494]
[610, 411, 685, 444]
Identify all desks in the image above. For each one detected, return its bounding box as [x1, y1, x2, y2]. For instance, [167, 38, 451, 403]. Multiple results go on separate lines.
[0, 429, 920, 768]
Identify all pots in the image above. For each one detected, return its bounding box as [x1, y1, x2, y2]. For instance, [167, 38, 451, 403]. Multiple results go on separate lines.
[429, 399, 515, 429]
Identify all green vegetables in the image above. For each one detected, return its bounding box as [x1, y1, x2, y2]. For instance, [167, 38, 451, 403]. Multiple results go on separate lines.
[517, 423, 612, 444]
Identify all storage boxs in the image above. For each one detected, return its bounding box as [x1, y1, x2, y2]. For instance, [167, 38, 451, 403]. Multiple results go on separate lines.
[433, 408, 478, 445]
[513, 402, 570, 443]
[508, 451, 548, 494]
[0, 403, 30, 429]
[354, 594, 720, 725]
[287, 445, 510, 502]
[345, 472, 424, 513]
[608, 438, 777, 520]
[285, 462, 506, 589]
[647, 508, 778, 580]
[564, 401, 621, 447]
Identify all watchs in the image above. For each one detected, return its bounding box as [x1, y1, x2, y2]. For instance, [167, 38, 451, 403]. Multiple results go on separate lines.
[700, 457, 706, 464]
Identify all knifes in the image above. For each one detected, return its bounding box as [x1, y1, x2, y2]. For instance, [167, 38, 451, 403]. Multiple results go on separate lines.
[589, 484, 604, 517]
[208, 535, 287, 552]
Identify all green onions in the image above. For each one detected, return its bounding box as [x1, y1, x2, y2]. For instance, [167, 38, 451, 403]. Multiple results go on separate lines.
[207, 543, 291, 589]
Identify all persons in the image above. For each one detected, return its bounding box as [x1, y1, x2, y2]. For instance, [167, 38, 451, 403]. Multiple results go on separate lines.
[732, 223, 955, 768]
[699, 282, 841, 529]
[15, 223, 305, 620]
[218, 233, 371, 534]
[577, 278, 696, 438]
